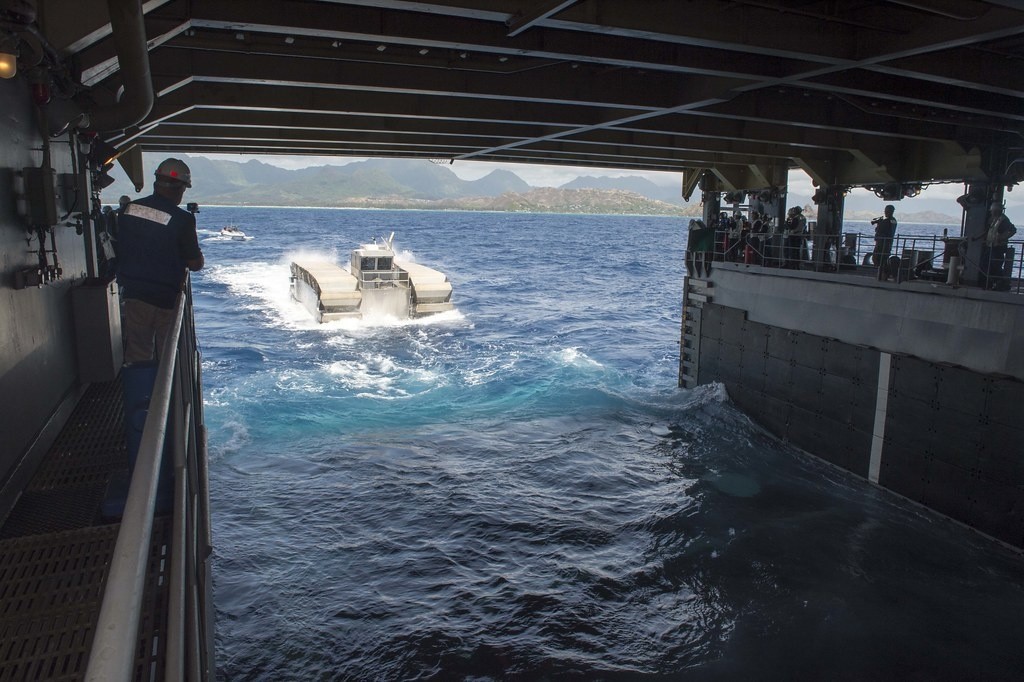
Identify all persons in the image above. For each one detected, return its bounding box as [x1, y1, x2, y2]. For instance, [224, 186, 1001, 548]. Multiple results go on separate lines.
[717, 210, 775, 265]
[982, 202, 1017, 290]
[782, 206, 806, 269]
[872, 205, 897, 281]
[101, 158, 204, 405]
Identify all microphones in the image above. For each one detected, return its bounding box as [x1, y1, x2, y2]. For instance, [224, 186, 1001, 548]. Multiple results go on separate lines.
[873, 218, 877, 220]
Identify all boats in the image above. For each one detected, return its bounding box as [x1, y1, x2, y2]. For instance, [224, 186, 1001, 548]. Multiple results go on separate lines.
[221, 225, 244, 240]
[290, 232, 453, 324]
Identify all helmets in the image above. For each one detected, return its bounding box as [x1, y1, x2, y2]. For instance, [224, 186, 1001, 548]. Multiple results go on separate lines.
[988, 202, 1006, 211]
[733, 210, 742, 216]
[102, 206, 112, 212]
[885, 205, 895, 212]
[793, 206, 802, 213]
[787, 207, 795, 215]
[155, 158, 192, 188]
[719, 213, 726, 218]
[119, 195, 130, 204]
[750, 210, 760, 216]
[762, 213, 771, 218]
[740, 215, 747, 220]
